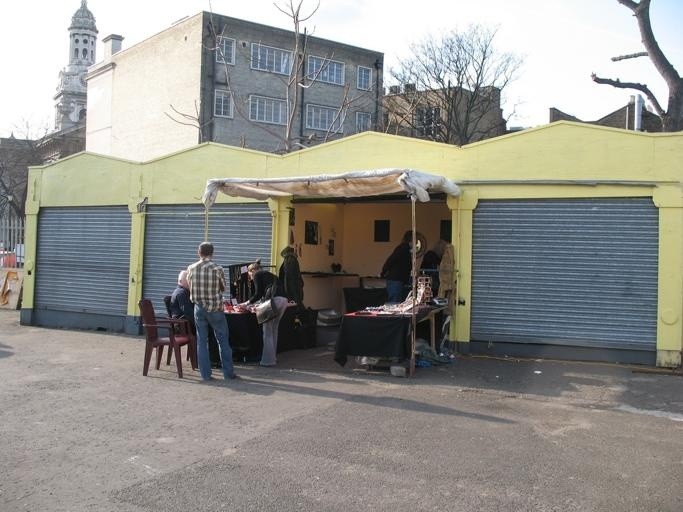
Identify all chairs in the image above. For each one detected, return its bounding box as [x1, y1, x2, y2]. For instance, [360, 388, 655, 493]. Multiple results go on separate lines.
[138, 299, 196, 378]
[164, 296, 199, 369]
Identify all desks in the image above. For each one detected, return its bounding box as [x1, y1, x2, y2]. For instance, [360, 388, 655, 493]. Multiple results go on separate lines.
[223, 303, 298, 363]
[334, 304, 450, 370]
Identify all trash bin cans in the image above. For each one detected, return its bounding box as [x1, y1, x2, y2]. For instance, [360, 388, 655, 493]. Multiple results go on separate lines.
[315, 309, 342, 346]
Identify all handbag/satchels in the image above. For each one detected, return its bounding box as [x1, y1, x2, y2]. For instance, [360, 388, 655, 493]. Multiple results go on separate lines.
[255, 299, 278, 325]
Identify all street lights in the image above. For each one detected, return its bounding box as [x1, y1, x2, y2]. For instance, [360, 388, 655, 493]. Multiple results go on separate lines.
[6, 191, 13, 251]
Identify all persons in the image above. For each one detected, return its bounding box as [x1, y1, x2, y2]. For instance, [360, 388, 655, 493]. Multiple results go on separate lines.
[378, 226, 453, 302]
[236, 258, 288, 366]
[169, 242, 236, 380]
